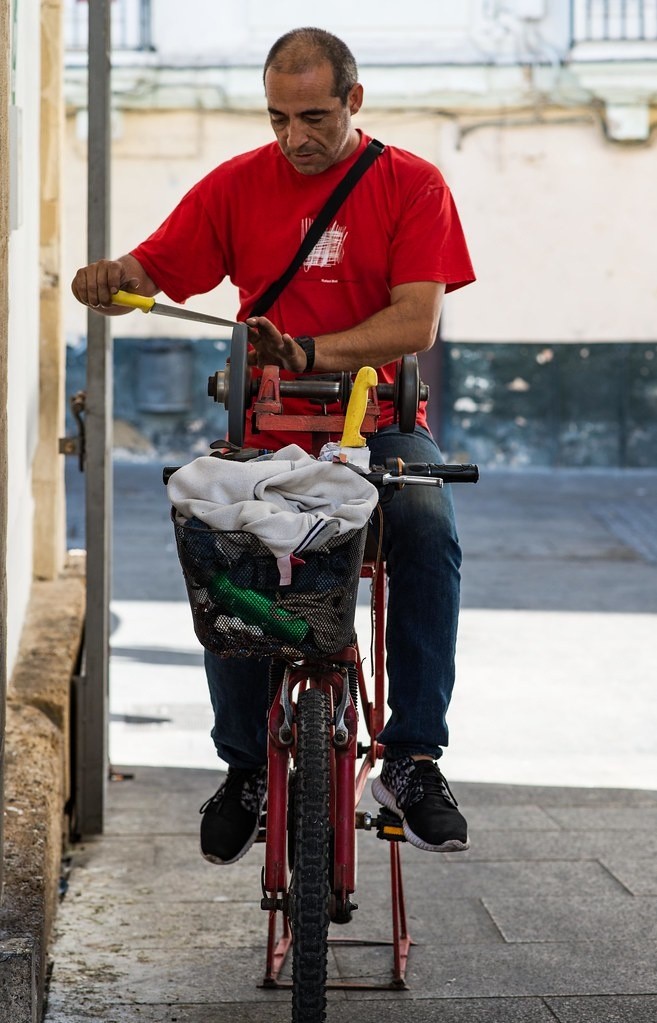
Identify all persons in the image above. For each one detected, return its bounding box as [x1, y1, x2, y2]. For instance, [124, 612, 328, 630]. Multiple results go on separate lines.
[71, 27, 478, 867]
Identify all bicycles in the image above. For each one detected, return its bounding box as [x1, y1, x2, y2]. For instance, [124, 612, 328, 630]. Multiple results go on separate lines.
[160, 456, 483, 1022]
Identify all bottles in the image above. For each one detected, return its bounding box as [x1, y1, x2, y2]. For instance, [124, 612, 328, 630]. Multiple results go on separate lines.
[209, 575, 309, 647]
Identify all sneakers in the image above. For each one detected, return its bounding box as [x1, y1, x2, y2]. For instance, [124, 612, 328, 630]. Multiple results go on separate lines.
[200, 763, 268, 864]
[371, 752, 470, 853]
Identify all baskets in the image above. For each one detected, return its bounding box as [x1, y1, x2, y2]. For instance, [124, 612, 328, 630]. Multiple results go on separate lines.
[171, 504, 367, 657]
